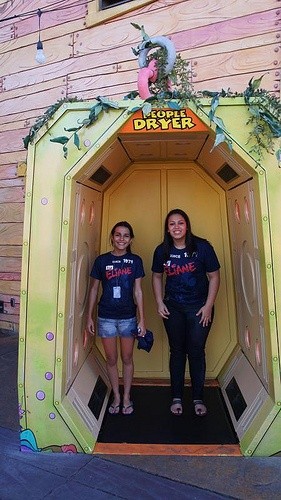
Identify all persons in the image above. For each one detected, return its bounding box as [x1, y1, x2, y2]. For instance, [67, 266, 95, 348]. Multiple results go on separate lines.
[151, 209, 221, 416]
[85, 221, 146, 415]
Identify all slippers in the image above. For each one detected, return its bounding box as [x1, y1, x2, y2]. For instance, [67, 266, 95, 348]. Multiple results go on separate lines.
[170, 398, 183, 416]
[193, 400, 208, 415]
[108, 402, 120, 414]
[121, 401, 133, 415]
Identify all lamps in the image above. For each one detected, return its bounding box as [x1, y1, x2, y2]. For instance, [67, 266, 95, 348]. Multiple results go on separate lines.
[34, 9, 47, 64]
